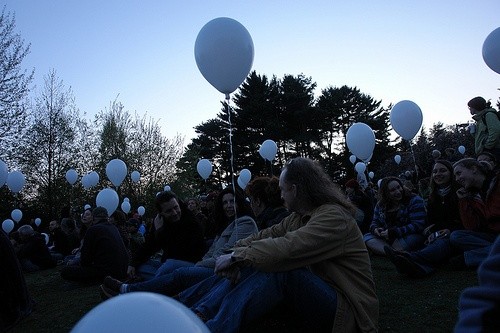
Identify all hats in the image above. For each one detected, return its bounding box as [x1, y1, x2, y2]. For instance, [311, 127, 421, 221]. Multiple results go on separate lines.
[125, 218, 140, 228]
[345, 179, 360, 188]
[201, 192, 220, 201]
[18, 225, 34, 234]
[92, 207, 108, 219]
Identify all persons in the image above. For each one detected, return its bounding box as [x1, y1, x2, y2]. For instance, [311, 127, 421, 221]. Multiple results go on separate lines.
[0, 96, 500, 333]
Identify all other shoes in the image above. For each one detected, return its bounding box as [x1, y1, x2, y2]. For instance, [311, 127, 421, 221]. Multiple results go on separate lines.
[383, 245, 410, 266]
[103, 276, 122, 292]
[394, 255, 413, 276]
[99, 286, 116, 300]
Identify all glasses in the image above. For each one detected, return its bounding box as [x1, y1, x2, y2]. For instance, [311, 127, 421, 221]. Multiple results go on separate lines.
[126, 223, 134, 228]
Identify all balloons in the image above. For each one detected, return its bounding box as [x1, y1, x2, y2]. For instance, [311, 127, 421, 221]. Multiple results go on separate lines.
[7, 171, 25, 192]
[0, 28, 500, 216]
[346, 123, 375, 160]
[35, 218, 41, 225]
[70, 292, 209, 333]
[11, 209, 22, 222]
[131, 171, 140, 183]
[88, 171, 99, 186]
[0, 159, 8, 188]
[459, 146, 465, 154]
[194, 17, 254, 94]
[84, 204, 90, 210]
[389, 100, 423, 140]
[106, 159, 127, 187]
[66, 170, 78, 185]
[82, 175, 91, 189]
[394, 155, 401, 164]
[2, 219, 14, 233]
[41, 233, 49, 244]
